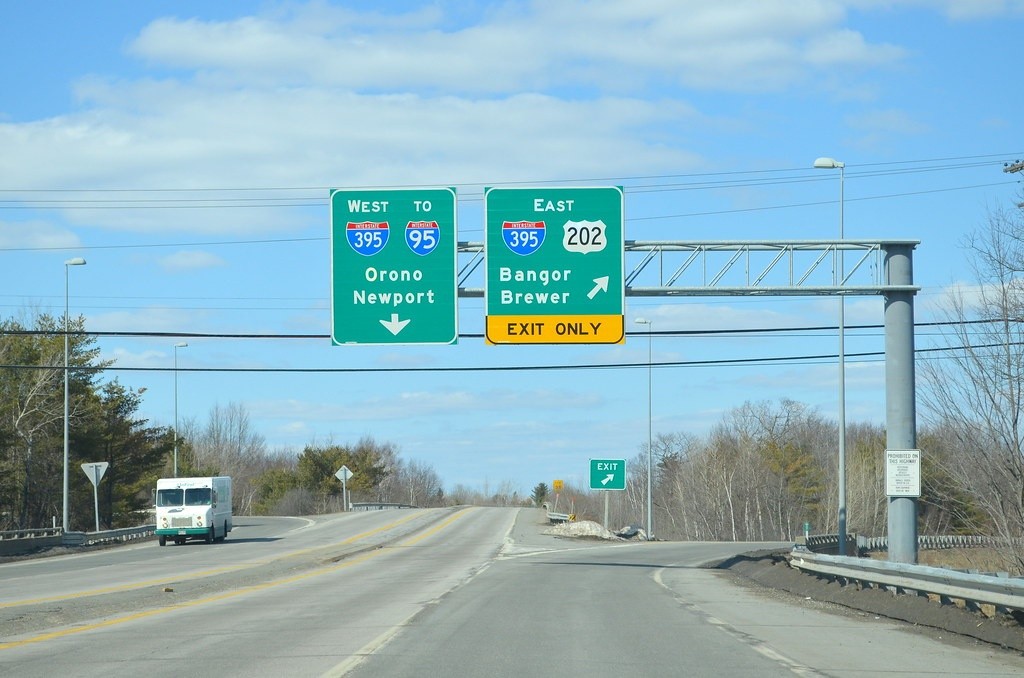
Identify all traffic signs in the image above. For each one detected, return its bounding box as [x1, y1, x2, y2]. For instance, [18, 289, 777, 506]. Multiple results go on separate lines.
[328, 187, 459, 346]
[589, 459, 626, 490]
[481, 185, 628, 344]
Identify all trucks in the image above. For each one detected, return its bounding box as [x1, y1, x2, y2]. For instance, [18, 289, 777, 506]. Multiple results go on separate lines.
[152, 476, 233, 547]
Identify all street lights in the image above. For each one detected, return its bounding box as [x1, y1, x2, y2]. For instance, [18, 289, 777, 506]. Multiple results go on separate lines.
[812, 157, 849, 552]
[174, 343, 191, 476]
[62, 257, 87, 535]
[635, 318, 655, 541]
